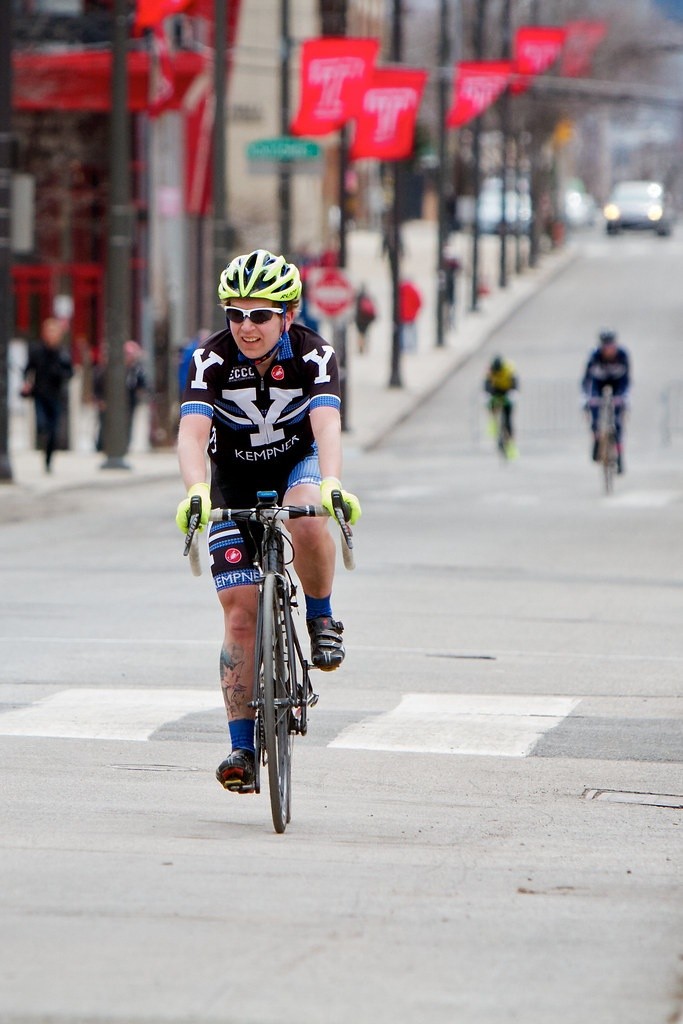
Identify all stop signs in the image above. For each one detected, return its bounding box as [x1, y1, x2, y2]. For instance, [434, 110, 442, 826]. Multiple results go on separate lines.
[315, 276, 349, 313]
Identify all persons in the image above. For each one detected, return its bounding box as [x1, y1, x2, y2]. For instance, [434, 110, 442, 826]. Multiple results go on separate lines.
[401, 279, 421, 353]
[355, 282, 374, 355]
[20, 318, 76, 476]
[484, 357, 519, 437]
[174, 249, 363, 795]
[582, 329, 629, 475]
[90, 339, 149, 473]
[176, 326, 212, 435]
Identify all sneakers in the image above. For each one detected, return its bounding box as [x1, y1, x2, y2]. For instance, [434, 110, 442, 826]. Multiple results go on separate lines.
[307, 616, 346, 666]
[216, 748, 255, 794]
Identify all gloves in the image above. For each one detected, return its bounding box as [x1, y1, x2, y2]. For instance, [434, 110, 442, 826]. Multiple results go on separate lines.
[320, 480, 362, 525]
[175, 483, 211, 534]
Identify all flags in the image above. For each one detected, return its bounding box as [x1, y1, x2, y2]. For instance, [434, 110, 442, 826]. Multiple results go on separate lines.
[291, 21, 608, 160]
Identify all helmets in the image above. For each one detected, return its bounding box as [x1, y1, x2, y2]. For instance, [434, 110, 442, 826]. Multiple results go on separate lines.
[599, 331, 616, 344]
[218, 249, 302, 301]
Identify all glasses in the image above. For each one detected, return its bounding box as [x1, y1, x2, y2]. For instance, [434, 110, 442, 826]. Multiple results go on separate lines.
[224, 304, 283, 323]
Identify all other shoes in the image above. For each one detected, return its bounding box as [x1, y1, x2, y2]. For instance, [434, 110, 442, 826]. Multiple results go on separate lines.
[616, 453, 622, 473]
[592, 440, 600, 461]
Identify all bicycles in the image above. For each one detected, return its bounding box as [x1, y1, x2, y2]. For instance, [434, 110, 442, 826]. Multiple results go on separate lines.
[585, 384, 621, 491]
[183, 490, 356, 835]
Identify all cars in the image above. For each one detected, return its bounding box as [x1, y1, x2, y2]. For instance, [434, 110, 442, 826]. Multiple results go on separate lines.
[605, 181, 670, 236]
[478, 177, 595, 237]
